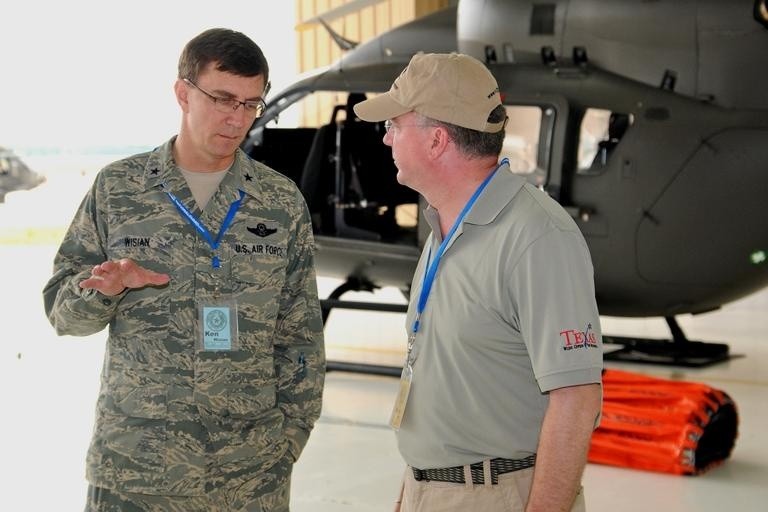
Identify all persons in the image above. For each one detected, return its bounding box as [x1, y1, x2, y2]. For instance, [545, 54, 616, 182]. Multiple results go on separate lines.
[44, 26, 328, 511]
[352, 50, 605, 511]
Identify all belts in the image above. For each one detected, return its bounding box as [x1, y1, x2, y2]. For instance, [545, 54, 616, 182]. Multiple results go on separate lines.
[405, 451, 537, 486]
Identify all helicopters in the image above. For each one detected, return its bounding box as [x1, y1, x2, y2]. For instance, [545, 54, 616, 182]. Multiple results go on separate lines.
[239, 0, 768, 378]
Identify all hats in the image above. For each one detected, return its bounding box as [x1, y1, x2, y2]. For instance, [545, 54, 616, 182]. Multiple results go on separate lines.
[351, 53, 510, 136]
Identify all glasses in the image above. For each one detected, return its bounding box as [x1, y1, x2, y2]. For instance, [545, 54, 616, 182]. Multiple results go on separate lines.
[183, 77, 267, 119]
[383, 120, 439, 137]
